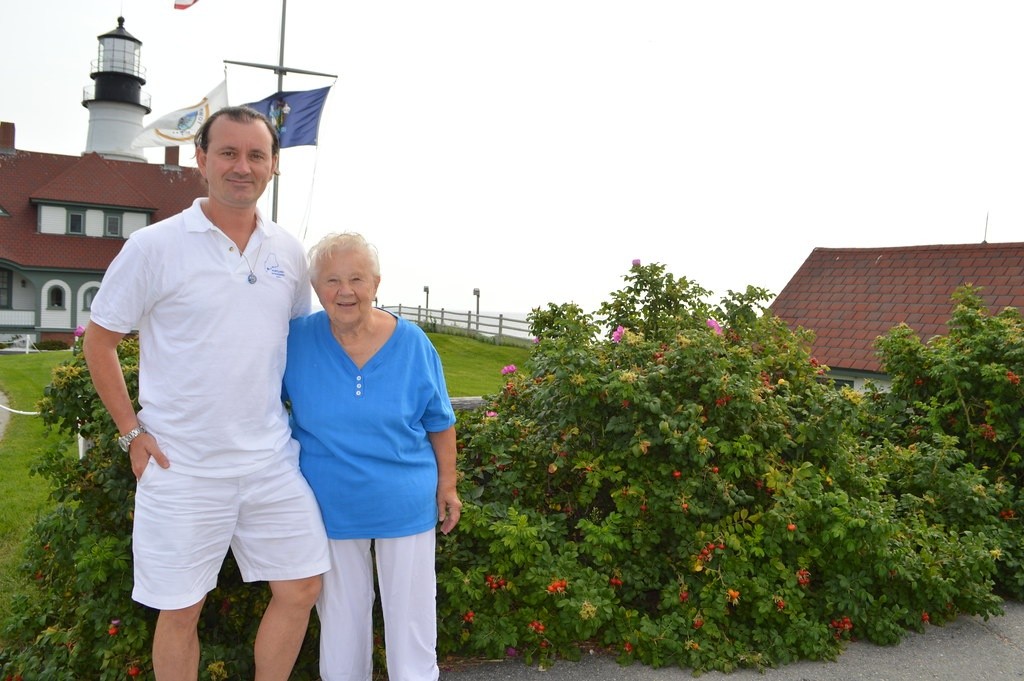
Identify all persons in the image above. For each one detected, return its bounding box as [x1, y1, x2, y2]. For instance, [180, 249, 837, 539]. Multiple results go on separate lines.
[280, 231, 463, 681]
[82, 104, 332, 681]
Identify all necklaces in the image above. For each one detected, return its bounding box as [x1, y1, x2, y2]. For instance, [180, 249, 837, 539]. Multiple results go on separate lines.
[202, 210, 263, 284]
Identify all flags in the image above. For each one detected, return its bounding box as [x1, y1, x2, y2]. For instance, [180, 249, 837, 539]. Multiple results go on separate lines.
[129, 79, 229, 155]
[237, 85, 332, 148]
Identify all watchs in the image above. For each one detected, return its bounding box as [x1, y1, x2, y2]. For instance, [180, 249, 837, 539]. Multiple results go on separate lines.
[117, 426, 145, 453]
